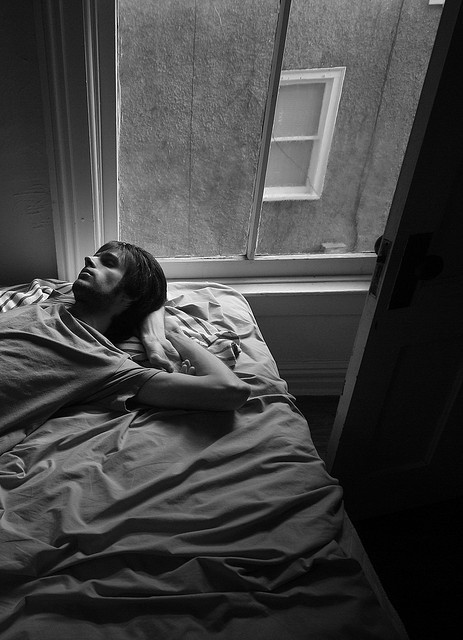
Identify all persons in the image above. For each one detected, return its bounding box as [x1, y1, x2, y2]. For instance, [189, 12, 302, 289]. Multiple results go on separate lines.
[1, 239, 252, 457]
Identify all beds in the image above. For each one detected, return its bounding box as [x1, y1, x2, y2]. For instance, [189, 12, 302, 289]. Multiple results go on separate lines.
[0, 278, 408, 639]
